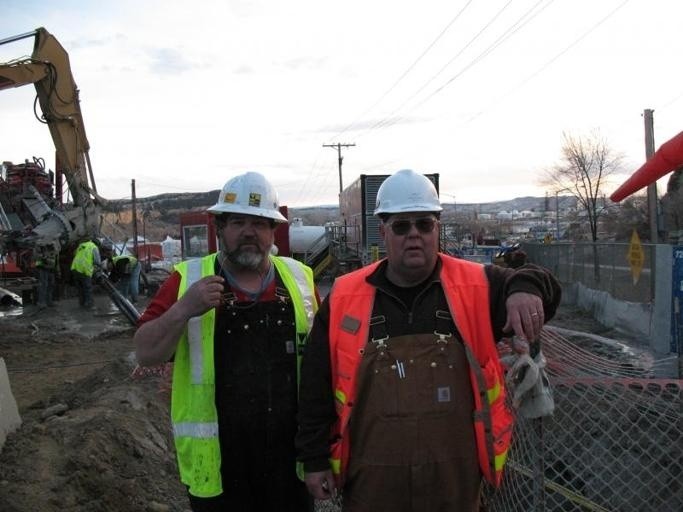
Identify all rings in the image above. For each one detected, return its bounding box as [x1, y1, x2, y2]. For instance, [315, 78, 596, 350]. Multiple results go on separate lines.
[530, 312, 539, 317]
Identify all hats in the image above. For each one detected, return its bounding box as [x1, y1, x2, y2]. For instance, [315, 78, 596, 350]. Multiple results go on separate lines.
[97, 236, 103, 246]
[372, 168, 444, 218]
[101, 259, 108, 272]
[205, 170, 289, 225]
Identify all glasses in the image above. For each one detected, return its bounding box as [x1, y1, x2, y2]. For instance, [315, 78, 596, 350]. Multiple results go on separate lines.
[384, 216, 441, 236]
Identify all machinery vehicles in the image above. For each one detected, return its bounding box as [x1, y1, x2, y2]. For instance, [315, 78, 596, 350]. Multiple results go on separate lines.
[0, 25, 115, 263]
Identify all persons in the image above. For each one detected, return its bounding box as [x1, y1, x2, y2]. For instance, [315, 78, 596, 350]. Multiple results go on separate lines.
[296, 168, 561, 512]
[501, 247, 526, 271]
[34, 245, 61, 309]
[101, 255, 141, 306]
[131, 170, 322, 512]
[70, 238, 101, 310]
